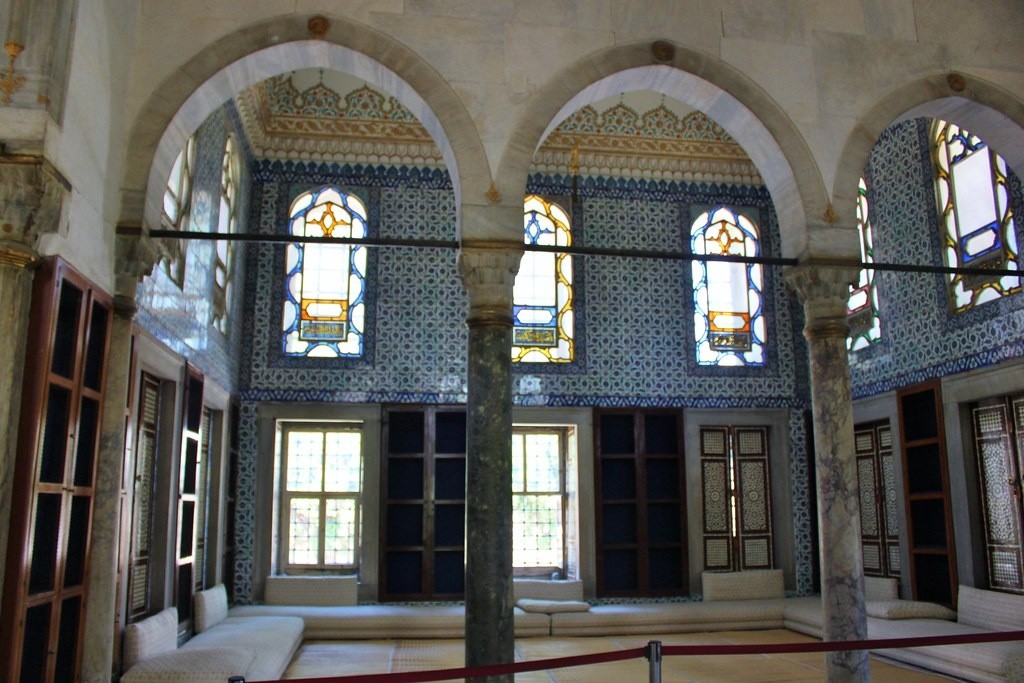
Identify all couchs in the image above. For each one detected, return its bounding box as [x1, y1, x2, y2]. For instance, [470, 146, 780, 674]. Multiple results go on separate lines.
[123, 584, 306, 683]
[229, 569, 1024, 682]
